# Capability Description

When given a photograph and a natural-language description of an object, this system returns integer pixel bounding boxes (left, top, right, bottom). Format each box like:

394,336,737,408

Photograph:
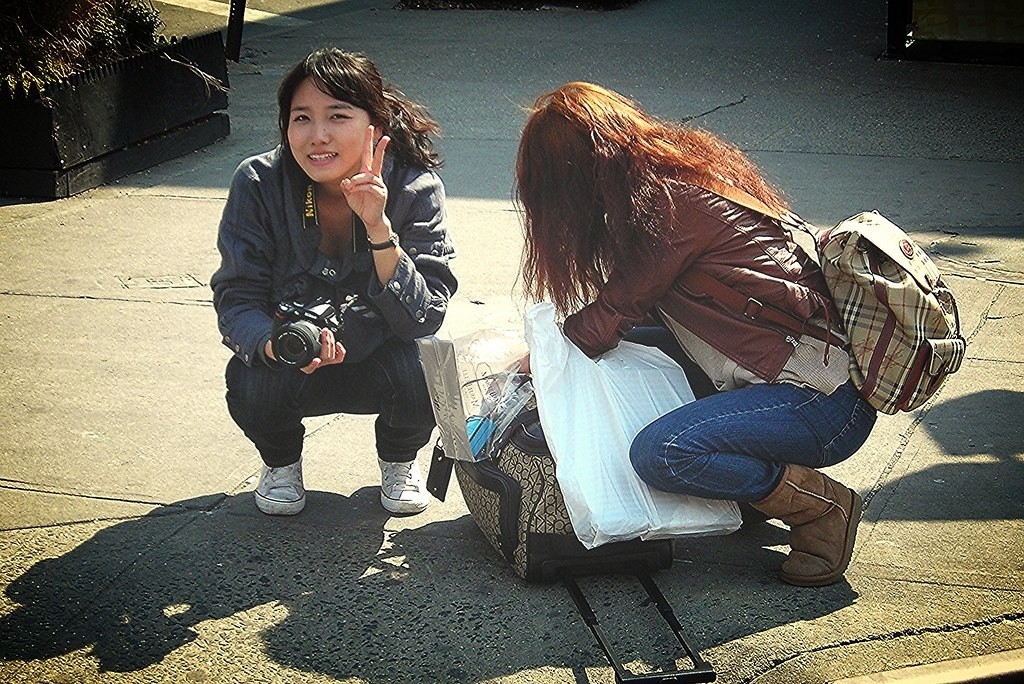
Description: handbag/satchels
524,298,743,551
415,324,533,461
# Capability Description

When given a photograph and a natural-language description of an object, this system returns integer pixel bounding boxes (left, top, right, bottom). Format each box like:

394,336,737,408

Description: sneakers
377,456,430,514
255,452,306,515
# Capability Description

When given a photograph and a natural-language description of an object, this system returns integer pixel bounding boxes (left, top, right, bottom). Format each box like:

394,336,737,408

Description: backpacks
658,167,967,415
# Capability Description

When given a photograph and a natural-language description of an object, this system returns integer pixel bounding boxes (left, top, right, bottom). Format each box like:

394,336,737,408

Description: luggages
453,360,673,582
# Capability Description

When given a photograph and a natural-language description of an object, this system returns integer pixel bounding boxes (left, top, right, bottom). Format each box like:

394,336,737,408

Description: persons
514,82,874,586
209,49,457,513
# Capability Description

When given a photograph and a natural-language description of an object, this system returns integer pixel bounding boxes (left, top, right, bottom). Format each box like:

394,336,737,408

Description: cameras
270,290,349,371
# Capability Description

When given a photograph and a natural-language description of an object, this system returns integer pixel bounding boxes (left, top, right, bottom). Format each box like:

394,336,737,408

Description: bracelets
367,232,399,250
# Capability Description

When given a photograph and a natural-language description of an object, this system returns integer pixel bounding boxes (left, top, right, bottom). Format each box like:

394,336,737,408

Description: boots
747,463,862,588
